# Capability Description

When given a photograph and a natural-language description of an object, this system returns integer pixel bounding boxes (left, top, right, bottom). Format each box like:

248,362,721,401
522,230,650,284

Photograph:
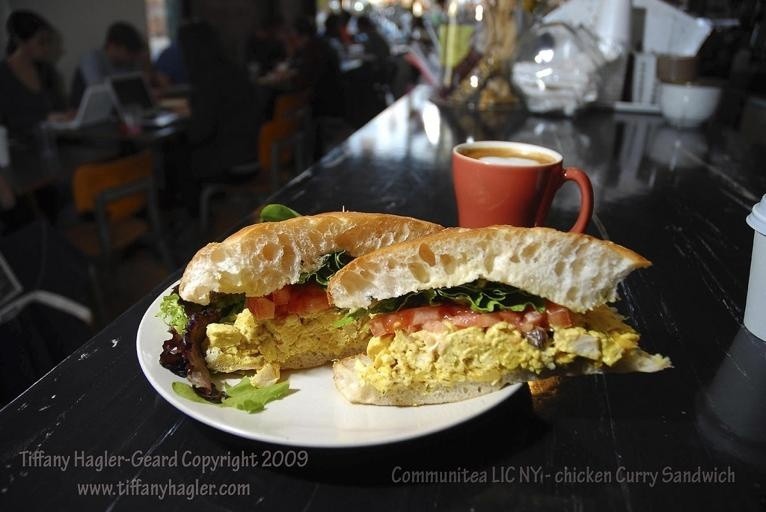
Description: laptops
72,84,111,123
105,73,154,119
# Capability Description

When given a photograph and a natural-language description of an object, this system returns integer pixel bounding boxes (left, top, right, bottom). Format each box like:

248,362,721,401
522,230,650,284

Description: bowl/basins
427,92,530,143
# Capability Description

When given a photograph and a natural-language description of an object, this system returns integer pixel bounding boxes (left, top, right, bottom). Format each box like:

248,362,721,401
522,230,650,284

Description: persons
0,9,436,409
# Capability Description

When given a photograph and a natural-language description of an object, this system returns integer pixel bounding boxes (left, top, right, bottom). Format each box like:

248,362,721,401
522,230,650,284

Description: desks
1,86,766,511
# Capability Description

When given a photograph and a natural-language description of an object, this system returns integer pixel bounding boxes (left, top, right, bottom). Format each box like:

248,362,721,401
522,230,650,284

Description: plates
133,277,528,450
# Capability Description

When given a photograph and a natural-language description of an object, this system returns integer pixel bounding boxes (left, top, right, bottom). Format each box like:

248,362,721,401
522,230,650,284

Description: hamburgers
157,205,675,415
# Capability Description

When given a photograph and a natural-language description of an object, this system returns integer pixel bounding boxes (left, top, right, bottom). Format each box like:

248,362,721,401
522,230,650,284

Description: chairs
61,150,168,310
197,86,311,243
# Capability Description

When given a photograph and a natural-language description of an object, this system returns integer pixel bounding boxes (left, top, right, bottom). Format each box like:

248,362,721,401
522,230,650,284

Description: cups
448,141,595,243
691,325,766,471
584,0,635,111
655,80,723,130
654,52,703,88
742,194,766,343
